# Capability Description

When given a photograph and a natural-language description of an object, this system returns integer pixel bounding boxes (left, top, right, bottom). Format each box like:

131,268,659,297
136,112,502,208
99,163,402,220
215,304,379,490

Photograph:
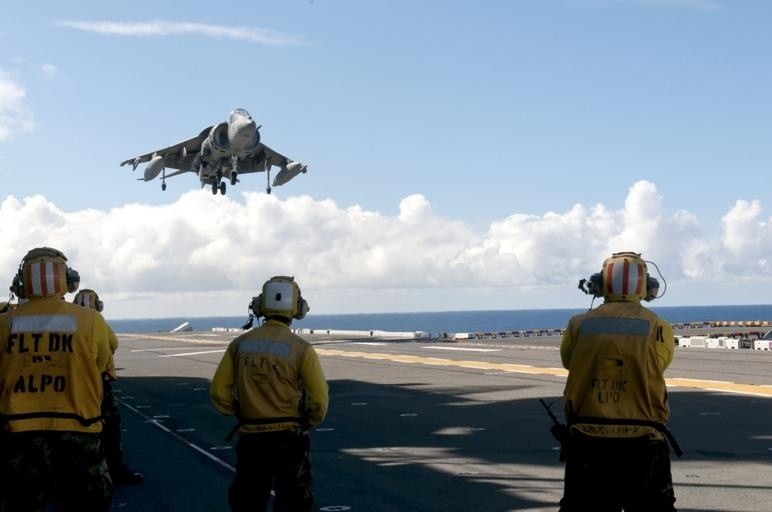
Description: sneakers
109,461,145,487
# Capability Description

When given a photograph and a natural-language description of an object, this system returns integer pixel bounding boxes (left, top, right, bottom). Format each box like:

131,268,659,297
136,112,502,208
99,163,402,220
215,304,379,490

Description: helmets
72,288,99,312
19,246,68,299
260,275,302,320
600,250,650,304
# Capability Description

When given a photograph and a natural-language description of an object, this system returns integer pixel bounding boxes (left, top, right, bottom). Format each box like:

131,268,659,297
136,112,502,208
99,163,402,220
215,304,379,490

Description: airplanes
120,108,308,195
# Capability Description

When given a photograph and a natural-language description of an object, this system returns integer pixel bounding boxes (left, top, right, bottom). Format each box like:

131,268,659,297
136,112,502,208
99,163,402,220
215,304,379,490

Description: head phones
248,275,310,320
9,247,80,298
589,251,660,303
74,288,103,312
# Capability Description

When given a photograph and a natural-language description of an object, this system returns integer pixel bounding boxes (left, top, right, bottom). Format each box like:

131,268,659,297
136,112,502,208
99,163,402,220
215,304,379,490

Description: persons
72,287,145,483
554,249,683,512
1,245,119,511
208,274,332,510
